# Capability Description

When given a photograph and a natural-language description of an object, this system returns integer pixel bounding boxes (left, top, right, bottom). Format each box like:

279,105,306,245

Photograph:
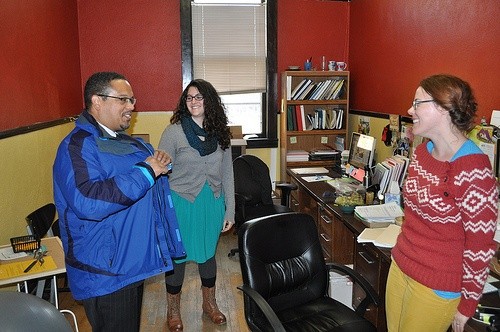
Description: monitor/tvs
347,131,376,171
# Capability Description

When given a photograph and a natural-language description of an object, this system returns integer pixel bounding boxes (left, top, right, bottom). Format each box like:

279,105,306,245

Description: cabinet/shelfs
286,164,403,332
280,70,349,197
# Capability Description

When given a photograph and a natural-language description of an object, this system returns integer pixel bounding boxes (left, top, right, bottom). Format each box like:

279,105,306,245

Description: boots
166,290,183,332
201,285,227,325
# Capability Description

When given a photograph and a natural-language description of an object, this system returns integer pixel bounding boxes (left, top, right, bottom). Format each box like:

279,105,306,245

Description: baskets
10,234,41,253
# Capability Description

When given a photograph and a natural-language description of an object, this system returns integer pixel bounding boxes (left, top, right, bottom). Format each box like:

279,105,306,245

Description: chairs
26,203,84,306
228,154,298,258
235,212,382,332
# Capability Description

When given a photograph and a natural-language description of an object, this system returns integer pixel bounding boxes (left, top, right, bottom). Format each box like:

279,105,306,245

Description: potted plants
335,192,364,214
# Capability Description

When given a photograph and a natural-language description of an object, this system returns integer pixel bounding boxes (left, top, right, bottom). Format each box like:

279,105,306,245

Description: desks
0,234,79,332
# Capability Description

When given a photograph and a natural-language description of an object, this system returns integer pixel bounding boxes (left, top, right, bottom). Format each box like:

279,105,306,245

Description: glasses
184,95,204,102
412,100,434,110
96,93,135,104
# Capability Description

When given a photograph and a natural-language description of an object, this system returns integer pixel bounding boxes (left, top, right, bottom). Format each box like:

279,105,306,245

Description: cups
304,62,312,71
336,61,347,71
328,61,336,72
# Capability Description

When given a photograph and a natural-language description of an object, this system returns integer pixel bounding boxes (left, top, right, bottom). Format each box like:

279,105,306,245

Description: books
286,76,409,196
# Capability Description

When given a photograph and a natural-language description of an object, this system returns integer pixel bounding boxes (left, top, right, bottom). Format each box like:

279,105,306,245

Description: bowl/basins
288,65,300,69
341,206,354,213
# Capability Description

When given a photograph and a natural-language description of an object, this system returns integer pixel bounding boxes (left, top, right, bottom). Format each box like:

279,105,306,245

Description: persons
384,74,499,332
158,80,237,332
52,72,186,332
